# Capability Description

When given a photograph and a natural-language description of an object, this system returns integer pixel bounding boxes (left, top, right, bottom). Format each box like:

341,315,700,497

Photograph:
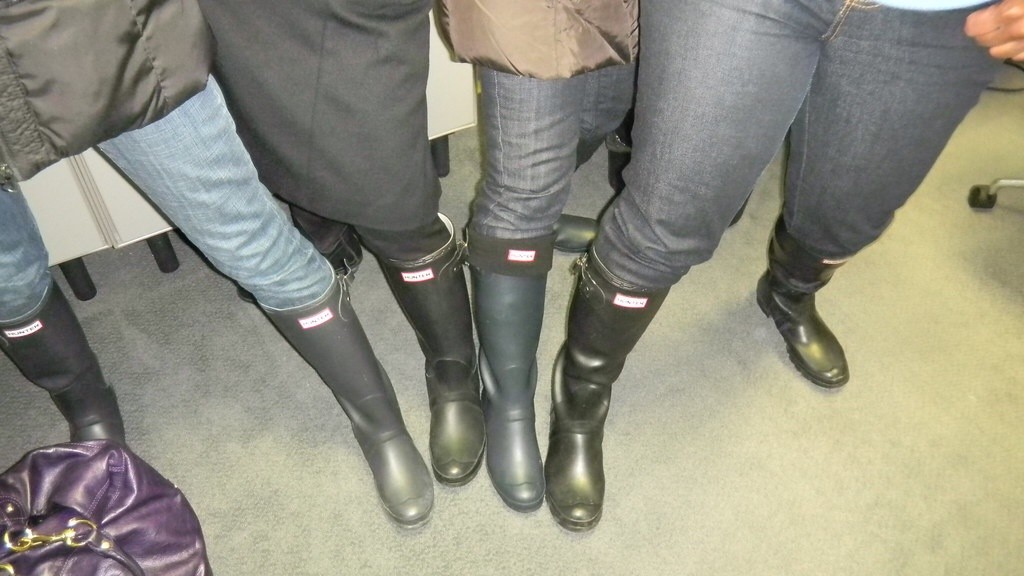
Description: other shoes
237,229,363,301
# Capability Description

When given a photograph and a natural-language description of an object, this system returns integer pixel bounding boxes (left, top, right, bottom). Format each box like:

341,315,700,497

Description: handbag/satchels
0,438,213,576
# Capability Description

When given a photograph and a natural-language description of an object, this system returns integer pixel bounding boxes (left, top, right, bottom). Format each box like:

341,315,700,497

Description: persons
545,0,1024,533
201,0,485,488
443,0,641,514
0,0,435,530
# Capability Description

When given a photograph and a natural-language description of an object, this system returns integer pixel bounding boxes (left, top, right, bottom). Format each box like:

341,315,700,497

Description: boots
552,138,605,252
0,272,124,444
462,226,558,513
374,211,485,487
257,255,435,530
544,240,671,532
756,201,849,388
